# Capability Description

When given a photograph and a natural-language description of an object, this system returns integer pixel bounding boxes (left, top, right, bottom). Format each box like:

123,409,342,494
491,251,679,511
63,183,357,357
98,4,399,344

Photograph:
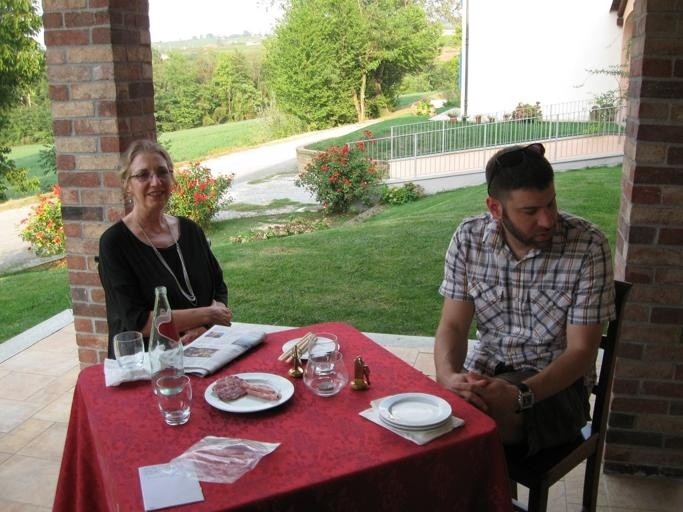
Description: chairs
511,280,633,510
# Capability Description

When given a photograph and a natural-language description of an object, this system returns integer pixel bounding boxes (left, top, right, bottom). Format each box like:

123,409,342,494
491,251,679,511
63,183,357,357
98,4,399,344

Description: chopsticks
278,332,316,363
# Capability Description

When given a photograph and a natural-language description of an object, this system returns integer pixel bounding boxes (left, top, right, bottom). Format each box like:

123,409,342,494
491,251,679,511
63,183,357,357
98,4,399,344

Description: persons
431,144,613,473
95,135,234,356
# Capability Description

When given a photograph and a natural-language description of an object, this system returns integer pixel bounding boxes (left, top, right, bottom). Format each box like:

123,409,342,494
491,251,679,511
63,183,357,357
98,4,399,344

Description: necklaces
129,208,199,306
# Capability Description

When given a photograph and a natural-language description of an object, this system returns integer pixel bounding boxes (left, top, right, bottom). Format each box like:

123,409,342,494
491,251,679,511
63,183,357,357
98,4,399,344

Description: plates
203,370,294,415
280,335,340,359
377,392,452,430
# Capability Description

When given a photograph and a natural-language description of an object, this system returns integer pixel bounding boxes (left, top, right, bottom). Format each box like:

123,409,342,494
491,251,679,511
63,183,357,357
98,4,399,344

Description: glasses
488,143,545,196
129,167,173,182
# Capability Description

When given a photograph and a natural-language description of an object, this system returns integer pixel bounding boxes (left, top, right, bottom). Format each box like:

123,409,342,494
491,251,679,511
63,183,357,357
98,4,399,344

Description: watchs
513,381,534,415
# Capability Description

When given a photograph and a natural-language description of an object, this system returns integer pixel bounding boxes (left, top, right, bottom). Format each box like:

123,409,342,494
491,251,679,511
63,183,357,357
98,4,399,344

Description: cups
307,331,339,354
302,350,349,398
155,374,195,428
111,328,145,371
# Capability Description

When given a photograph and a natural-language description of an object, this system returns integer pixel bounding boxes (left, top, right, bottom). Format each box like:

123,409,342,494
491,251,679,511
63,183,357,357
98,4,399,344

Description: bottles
148,284,184,397
